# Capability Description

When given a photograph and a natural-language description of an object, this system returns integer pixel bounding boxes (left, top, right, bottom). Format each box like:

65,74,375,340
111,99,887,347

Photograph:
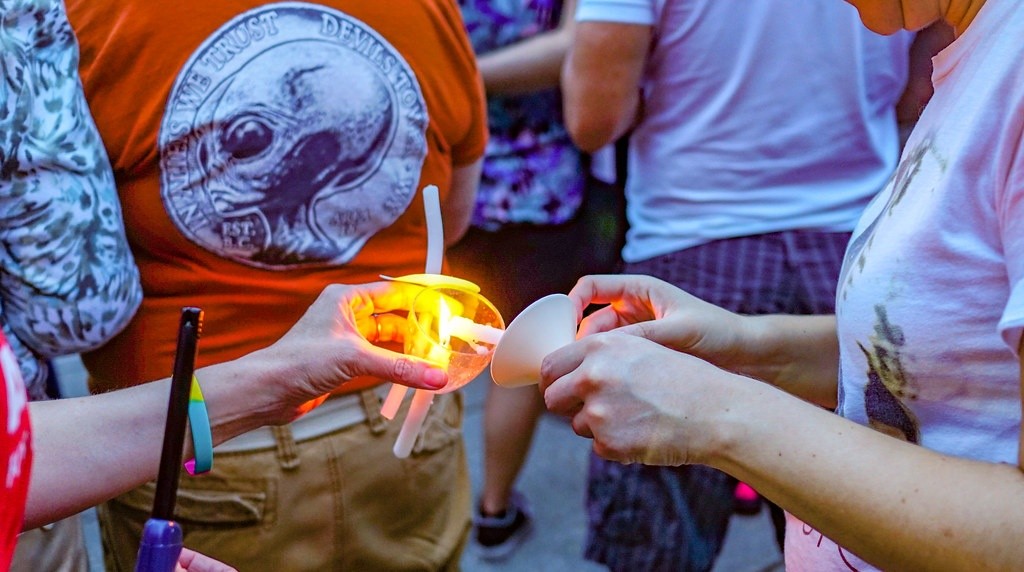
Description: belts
211,379,416,453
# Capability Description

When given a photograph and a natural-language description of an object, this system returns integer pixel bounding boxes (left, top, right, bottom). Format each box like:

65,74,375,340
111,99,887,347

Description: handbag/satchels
575,131,630,278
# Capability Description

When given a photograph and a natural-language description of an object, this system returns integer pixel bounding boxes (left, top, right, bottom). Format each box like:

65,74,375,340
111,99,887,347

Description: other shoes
737,495,761,515
474,490,535,559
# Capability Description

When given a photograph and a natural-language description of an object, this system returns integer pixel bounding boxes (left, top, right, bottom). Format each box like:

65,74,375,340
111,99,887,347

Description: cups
403,284,505,394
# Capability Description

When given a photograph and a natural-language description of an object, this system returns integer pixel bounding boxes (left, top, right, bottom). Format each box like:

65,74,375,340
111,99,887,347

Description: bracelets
182,366,215,478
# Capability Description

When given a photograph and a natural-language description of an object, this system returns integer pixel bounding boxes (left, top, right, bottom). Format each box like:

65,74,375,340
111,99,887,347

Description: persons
0,0,954,572
539,0,1024,572
